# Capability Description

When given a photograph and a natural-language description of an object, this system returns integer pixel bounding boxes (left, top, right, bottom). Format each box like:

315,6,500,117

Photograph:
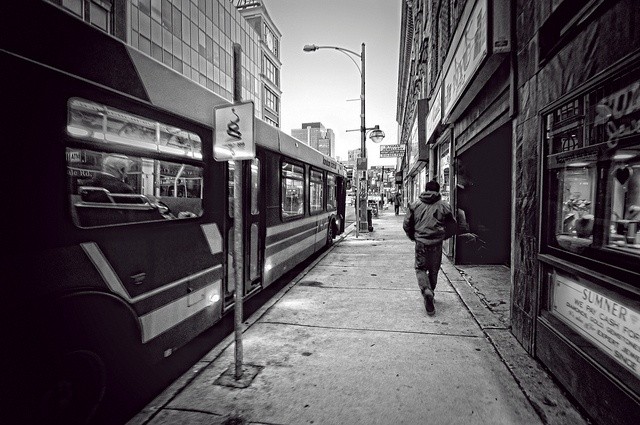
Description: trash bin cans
358,207,372,232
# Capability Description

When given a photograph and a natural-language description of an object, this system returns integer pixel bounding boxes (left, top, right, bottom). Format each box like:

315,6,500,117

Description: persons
84,154,144,203
392,194,402,214
403,181,456,315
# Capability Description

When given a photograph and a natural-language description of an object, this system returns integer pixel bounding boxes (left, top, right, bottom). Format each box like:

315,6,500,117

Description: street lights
303,41,386,234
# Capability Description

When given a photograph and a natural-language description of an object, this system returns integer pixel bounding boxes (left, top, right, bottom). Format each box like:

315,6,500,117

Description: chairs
73,187,166,227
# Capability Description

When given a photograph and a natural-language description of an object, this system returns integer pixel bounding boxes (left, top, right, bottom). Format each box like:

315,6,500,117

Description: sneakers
424,290,436,316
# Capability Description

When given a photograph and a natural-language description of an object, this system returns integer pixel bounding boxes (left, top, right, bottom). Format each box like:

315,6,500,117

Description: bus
0,0,353,424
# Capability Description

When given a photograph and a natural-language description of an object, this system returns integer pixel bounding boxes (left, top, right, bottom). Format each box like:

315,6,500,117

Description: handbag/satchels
398,201,402,206
